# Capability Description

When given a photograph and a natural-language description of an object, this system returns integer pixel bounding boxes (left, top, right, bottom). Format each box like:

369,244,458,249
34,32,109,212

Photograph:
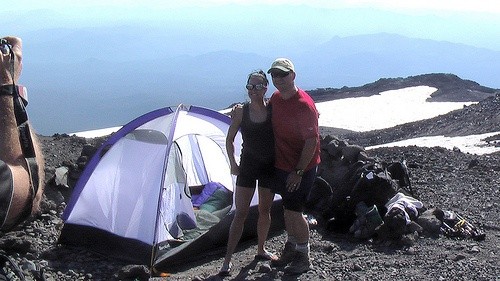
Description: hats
267,58,295,74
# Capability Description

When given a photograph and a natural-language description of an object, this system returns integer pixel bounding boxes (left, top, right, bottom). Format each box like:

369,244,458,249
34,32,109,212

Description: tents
57,104,285,268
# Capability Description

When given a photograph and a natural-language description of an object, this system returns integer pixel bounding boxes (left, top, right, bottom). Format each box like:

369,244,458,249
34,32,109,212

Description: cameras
0,39,12,54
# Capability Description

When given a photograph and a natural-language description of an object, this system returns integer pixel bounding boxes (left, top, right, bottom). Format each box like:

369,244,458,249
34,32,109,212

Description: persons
219,70,320,275
0,37,45,232
230,58,320,274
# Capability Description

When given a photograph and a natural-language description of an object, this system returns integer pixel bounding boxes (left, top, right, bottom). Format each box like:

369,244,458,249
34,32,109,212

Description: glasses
246,83,268,90
271,72,289,77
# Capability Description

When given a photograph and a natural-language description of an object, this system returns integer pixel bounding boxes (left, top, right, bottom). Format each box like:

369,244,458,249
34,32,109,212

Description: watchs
293,167,304,177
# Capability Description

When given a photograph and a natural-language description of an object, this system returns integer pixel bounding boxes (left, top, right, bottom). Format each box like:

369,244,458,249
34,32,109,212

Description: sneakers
272,242,312,276
255,249,279,262
219,262,232,276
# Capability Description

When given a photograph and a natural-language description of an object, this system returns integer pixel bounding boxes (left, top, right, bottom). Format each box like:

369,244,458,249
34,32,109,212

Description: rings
293,183,297,187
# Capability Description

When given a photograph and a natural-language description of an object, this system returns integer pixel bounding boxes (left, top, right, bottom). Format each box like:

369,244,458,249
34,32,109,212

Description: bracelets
0,84,28,107
